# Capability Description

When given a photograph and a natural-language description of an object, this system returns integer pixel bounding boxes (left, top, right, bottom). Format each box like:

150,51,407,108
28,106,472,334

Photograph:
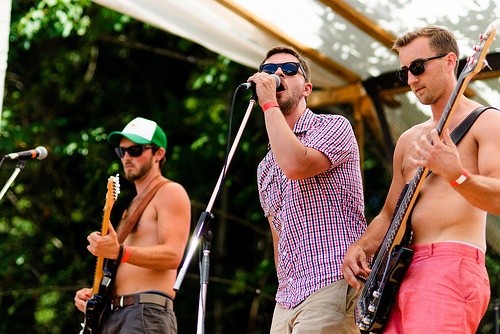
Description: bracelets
449,168,469,186
117,244,131,262
262,102,279,112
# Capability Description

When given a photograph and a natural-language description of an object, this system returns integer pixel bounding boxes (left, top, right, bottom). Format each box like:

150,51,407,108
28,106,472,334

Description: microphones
8,146,48,160
240,74,281,89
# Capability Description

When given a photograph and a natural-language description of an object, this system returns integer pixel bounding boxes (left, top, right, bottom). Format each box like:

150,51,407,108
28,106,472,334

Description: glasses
115,144,158,158
399,55,459,82
260,62,307,80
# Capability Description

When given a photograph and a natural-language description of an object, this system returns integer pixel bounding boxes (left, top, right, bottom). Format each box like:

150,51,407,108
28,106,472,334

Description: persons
246,46,374,334
73,116,191,334
342,26,500,334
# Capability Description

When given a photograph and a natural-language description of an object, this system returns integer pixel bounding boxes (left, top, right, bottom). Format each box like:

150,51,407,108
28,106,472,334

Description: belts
108,294,173,310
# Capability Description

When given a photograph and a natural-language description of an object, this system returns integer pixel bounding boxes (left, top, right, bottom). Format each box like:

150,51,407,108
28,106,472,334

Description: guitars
79,174,123,334
354,19,500,334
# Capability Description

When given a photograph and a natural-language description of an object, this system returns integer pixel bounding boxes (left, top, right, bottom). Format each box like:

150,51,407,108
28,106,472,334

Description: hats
108,117,167,150
